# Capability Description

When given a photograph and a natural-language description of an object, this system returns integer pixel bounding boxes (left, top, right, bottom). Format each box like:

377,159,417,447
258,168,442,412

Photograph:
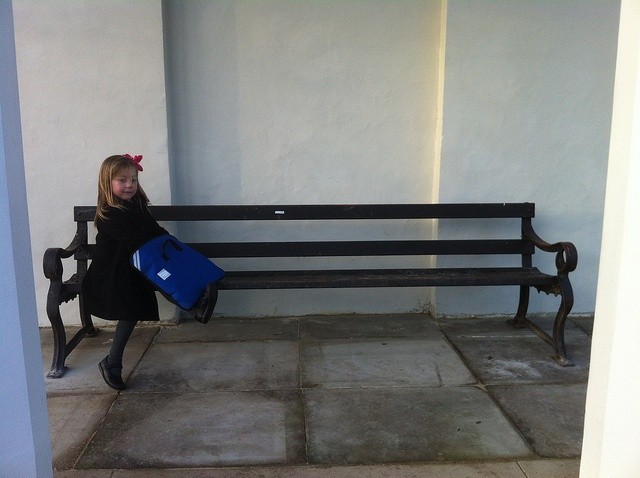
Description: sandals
193,281,219,324
99,354,126,390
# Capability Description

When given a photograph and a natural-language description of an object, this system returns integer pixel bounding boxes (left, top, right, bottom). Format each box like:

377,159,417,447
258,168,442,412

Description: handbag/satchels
129,233,225,310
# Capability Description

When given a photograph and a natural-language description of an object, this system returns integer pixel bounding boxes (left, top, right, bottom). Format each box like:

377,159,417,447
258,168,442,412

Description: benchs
43,202,578,378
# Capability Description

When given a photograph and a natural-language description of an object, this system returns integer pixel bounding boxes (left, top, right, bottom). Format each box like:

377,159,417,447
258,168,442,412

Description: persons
80,153,218,391
